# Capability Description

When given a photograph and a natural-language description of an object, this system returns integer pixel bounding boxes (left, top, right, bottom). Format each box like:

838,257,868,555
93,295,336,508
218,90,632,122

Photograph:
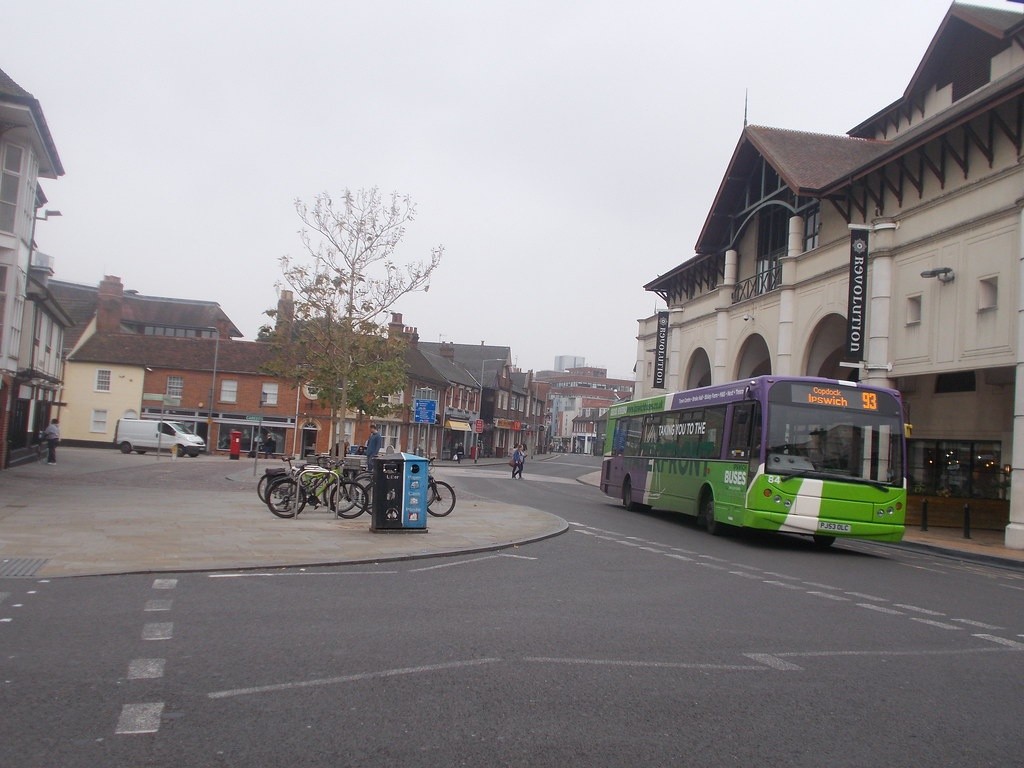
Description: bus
598,375,914,548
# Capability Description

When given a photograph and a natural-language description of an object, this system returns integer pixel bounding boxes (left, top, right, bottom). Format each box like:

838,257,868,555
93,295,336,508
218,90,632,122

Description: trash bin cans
370,452,429,533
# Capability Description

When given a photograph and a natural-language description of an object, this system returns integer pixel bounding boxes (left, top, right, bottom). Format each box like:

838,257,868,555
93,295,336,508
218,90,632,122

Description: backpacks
508,450,520,467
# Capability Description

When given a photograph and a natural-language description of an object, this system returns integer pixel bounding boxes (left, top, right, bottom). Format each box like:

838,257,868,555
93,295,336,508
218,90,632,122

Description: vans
115,418,206,458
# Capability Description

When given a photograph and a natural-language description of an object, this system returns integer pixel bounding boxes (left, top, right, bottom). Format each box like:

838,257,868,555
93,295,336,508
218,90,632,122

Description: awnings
445,419,471,431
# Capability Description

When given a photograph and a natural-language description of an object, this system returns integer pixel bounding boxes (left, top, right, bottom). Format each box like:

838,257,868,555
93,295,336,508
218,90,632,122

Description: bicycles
257,450,456,519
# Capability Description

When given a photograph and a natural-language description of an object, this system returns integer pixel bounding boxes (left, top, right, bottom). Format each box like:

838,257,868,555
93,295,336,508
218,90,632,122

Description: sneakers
47,460,56,465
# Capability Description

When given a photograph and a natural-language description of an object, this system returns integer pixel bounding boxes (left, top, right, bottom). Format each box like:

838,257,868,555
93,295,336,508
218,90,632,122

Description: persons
264,433,273,459
44,419,59,466
513,444,528,479
512,442,519,479
355,446,366,455
366,424,381,470
452,442,464,464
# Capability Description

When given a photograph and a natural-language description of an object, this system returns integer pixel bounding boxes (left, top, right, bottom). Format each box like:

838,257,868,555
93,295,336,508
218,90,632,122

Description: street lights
205,325,220,453
473,358,509,464
